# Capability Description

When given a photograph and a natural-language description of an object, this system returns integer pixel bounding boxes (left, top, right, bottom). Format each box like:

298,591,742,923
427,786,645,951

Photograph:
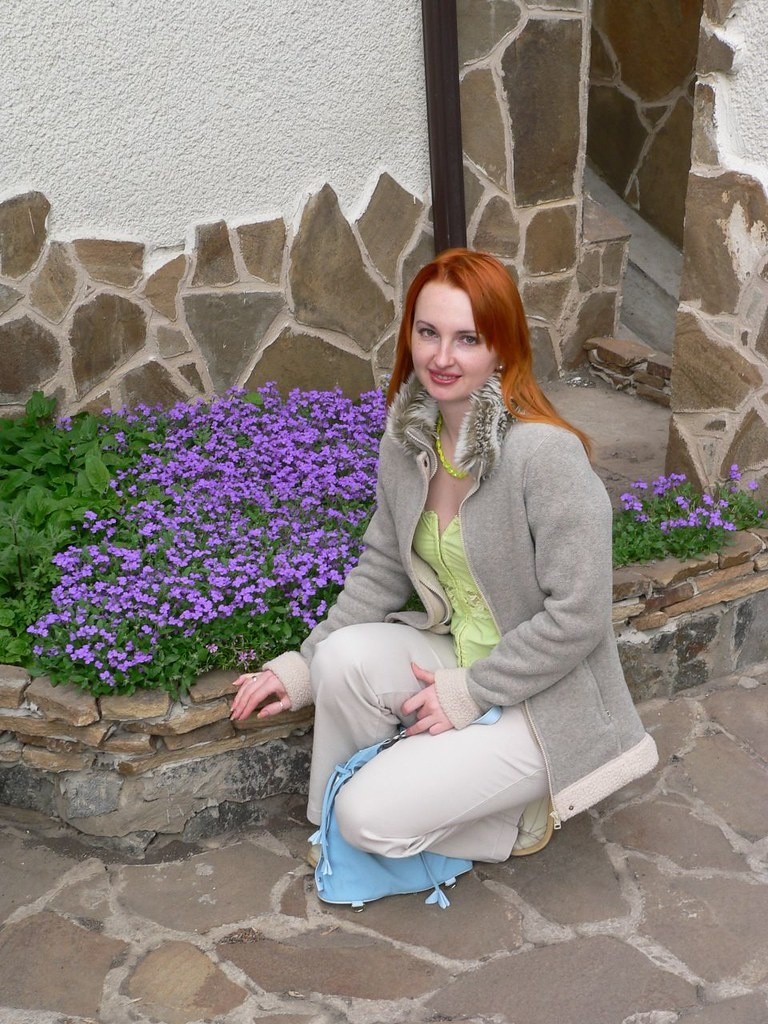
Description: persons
228,248,660,869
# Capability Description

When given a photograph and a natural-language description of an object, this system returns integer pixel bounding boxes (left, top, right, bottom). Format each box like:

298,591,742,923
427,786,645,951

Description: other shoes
510,795,555,856
308,843,322,868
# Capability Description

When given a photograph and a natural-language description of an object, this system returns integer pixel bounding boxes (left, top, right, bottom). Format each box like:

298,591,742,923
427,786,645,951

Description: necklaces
432,414,474,479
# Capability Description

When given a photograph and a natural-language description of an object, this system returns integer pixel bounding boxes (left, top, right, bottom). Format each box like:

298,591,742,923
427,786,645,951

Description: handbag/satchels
308,737,474,913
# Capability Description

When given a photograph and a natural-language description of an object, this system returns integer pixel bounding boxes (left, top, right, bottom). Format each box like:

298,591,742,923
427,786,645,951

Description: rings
251,675,259,682
279,699,286,711
